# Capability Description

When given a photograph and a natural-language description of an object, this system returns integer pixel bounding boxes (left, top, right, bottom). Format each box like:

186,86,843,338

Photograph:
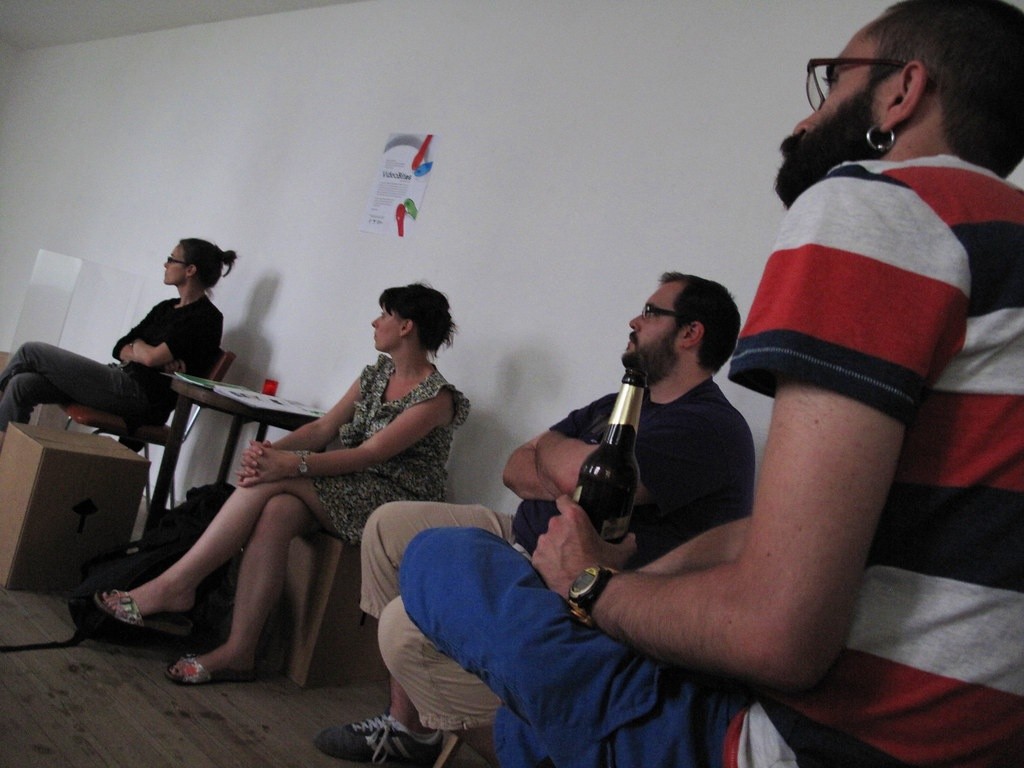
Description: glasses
641,303,676,319
806,57,937,112
167,257,199,273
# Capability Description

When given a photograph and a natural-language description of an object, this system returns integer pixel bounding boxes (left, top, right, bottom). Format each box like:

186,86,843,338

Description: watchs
298,452,308,478
568,563,622,628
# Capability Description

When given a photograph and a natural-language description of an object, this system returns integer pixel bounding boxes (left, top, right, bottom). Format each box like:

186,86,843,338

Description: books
174,371,326,418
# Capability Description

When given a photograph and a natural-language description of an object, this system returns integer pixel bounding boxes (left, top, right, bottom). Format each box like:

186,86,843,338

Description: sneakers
314,713,443,768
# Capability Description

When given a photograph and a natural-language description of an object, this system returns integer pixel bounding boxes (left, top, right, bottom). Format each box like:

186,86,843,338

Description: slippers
164,653,259,685
94,589,194,634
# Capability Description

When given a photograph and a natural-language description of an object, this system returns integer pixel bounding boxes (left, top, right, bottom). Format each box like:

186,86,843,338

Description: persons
0,238,237,447
94,283,470,684
315,271,756,768
398,0,1024,768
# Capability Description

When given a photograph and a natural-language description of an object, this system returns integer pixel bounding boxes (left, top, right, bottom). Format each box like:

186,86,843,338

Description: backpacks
158,481,236,553
70,519,236,651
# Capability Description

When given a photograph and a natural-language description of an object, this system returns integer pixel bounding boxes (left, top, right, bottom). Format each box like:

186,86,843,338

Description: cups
262,378,279,396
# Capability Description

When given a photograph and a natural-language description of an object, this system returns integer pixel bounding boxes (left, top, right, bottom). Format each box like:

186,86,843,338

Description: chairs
59,347,237,514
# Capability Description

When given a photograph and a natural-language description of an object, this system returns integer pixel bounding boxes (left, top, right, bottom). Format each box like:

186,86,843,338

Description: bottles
569,369,651,545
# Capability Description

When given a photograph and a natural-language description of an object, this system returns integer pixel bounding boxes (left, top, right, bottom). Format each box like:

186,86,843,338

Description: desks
170,378,319,485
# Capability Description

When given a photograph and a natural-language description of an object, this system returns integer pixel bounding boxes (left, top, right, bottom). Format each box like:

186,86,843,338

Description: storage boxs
0,420,152,592
215,526,389,687
